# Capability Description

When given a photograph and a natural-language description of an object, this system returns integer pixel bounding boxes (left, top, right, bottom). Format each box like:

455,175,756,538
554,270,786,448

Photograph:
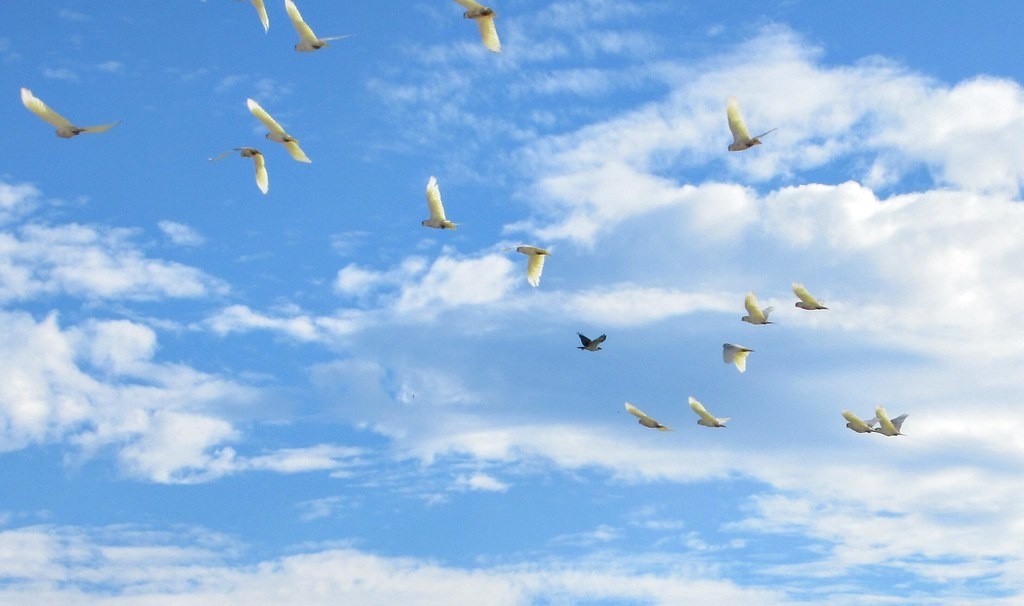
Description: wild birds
792,279,830,311
284,0,353,53
726,95,778,151
624,402,677,432
450,0,502,54
500,244,552,288
722,343,756,374
742,286,776,325
576,331,608,352
841,403,909,438
421,175,457,231
207,97,312,195
688,395,732,429
250,0,270,34
20,87,123,138
